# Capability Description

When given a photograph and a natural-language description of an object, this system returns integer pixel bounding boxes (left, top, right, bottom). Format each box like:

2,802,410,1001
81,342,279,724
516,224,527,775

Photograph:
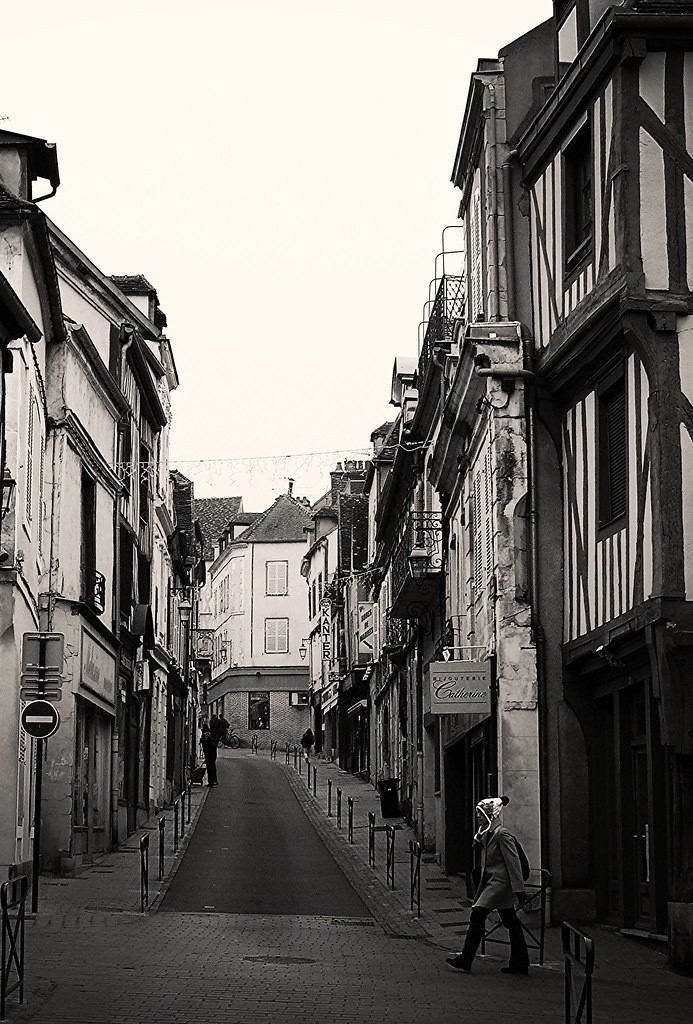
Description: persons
300,728,314,764
445,796,530,975
200,714,229,787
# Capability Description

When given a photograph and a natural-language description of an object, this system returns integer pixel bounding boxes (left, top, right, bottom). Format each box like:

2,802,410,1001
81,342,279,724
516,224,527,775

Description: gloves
515,891,528,905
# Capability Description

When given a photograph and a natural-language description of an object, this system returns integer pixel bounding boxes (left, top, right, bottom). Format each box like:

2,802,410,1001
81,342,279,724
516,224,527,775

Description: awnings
421,657,491,732
347,700,367,716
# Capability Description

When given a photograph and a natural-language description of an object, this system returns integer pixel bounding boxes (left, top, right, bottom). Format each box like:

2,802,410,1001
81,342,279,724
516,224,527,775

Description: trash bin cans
377,777,401,819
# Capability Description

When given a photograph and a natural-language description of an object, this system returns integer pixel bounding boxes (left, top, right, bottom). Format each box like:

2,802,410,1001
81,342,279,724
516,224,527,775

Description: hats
474,796,510,842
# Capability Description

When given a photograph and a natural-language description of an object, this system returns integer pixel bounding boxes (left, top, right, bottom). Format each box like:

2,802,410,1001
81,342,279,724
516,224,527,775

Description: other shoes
446,956,471,971
501,966,529,974
305,755,308,759
225,745,228,748
205,782,218,787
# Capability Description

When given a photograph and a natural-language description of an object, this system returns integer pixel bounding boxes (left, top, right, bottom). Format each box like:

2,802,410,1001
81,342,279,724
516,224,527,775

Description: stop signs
20,699,58,740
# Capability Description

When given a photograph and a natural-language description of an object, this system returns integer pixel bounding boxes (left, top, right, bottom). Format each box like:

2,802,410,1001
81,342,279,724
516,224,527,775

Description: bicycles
218,729,241,750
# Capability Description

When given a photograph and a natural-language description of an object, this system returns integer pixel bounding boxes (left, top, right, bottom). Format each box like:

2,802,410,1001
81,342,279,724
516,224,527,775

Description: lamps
219,641,229,660
298,638,310,660
406,526,448,586
169,588,193,628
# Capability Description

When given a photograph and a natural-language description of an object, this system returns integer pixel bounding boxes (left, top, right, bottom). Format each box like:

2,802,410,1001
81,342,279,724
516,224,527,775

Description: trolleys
190,761,207,786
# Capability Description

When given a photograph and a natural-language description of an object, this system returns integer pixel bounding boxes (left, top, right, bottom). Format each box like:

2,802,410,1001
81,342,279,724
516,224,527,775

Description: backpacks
498,828,530,881
